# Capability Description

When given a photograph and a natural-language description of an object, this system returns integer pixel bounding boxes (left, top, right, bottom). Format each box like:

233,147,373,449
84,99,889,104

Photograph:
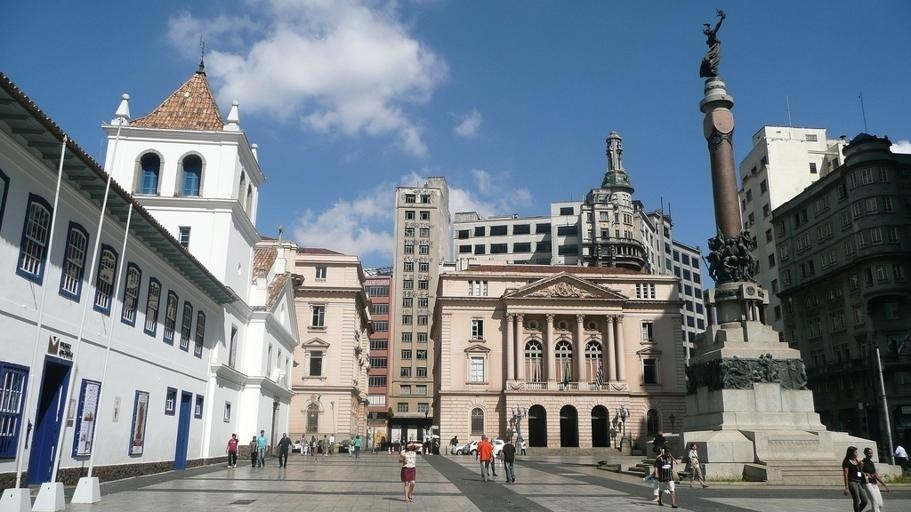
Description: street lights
372,411,378,454
616,404,633,456
331,399,337,445
511,404,527,455
859,400,871,438
425,408,430,436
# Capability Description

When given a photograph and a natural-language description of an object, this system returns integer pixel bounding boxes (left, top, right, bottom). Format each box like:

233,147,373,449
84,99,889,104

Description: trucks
880,398,911,446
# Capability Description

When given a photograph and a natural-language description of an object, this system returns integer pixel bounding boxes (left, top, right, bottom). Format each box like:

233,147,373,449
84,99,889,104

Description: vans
464,439,506,455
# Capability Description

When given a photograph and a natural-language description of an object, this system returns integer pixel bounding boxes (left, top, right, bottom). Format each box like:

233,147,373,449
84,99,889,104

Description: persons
298,433,363,465
276,433,295,468
256,430,269,468
687,442,710,489
397,440,424,505
249,436,258,467
858,446,889,512
645,445,680,509
893,443,909,472
474,433,529,483
424,438,440,454
448,435,459,455
698,17,723,77
224,432,239,468
705,228,756,280
842,446,869,512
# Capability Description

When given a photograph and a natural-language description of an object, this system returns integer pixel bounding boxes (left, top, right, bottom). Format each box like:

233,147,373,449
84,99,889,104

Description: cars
318,439,331,451
407,440,424,452
456,441,466,454
339,439,351,452
293,440,308,451
